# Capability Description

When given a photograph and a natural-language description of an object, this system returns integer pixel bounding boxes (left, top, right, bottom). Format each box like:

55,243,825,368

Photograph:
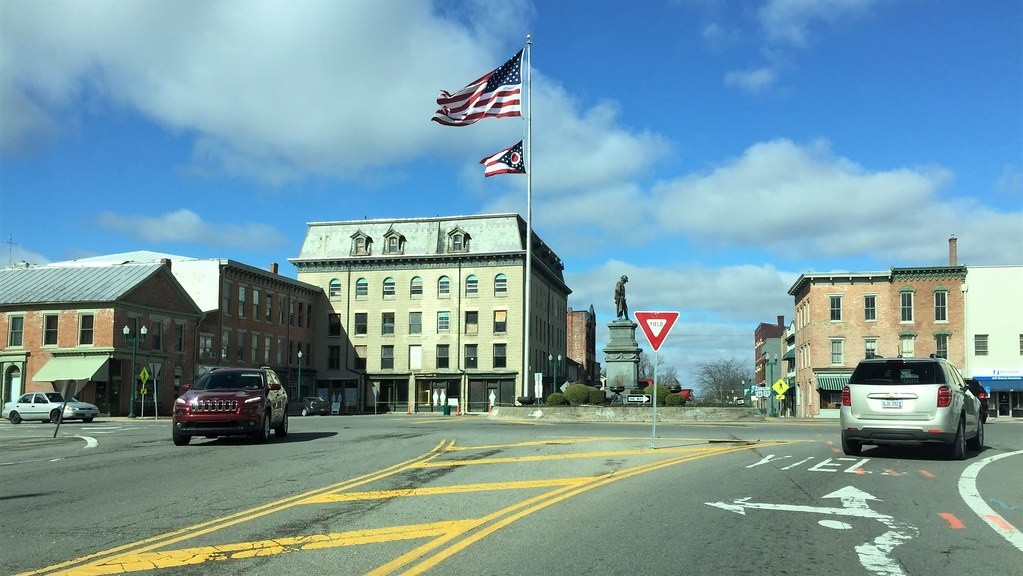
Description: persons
614,274,630,320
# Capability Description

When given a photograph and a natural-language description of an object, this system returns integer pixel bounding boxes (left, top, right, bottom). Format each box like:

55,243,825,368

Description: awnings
781,347,795,360
817,376,851,390
32,354,111,382
978,381,1023,393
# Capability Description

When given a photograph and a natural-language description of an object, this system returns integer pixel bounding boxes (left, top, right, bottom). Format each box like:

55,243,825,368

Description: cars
2,391,101,425
287,396,330,416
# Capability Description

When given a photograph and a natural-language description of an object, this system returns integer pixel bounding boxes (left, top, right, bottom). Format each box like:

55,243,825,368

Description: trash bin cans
442,402,451,416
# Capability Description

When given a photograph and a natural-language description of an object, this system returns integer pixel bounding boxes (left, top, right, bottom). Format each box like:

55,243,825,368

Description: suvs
838,353,985,461
171,366,290,446
963,378,989,423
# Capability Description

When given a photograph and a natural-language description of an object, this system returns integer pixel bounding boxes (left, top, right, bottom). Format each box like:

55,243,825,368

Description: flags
479,138,527,177
430,47,526,127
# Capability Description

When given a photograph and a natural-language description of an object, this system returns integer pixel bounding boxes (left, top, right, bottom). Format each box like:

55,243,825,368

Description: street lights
741,380,746,406
731,389,734,407
763,352,779,417
749,375,753,407
122,325,148,420
297,350,304,401
549,354,561,393
